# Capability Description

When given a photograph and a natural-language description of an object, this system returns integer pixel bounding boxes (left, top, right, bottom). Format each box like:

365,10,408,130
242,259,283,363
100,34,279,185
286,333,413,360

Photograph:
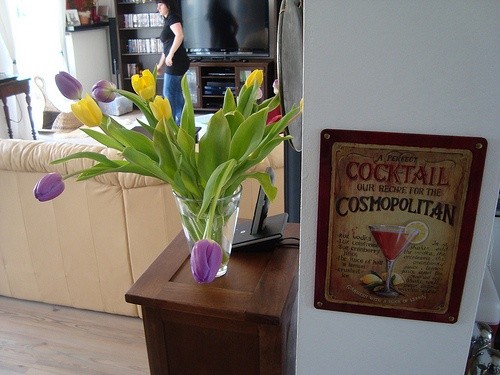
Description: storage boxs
98,95,134,116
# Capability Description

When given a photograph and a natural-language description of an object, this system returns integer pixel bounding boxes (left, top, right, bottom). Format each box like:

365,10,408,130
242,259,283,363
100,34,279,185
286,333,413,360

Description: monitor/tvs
178,0,277,62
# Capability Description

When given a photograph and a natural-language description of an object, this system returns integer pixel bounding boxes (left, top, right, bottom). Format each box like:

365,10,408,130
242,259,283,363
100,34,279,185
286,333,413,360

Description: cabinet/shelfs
124,218,300,375
114,0,164,95
187,59,272,112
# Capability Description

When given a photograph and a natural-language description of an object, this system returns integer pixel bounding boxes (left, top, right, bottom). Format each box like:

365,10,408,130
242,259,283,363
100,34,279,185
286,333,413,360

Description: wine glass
369,224,421,297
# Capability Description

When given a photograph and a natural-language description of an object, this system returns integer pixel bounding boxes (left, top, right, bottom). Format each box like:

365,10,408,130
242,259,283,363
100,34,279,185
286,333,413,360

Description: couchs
0,137,283,317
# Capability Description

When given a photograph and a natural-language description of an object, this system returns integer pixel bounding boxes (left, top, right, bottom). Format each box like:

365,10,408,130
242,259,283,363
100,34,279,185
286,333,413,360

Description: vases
172,185,243,278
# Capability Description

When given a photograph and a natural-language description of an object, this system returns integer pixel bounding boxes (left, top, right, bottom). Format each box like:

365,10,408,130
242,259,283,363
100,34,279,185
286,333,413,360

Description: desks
0,78,37,140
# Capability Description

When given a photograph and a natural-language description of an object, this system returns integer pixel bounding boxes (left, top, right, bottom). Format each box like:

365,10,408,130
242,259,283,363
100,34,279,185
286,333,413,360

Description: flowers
33,69,303,283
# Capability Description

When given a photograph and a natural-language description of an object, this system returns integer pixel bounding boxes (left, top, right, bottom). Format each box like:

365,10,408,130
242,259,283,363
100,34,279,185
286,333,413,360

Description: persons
155,0,190,125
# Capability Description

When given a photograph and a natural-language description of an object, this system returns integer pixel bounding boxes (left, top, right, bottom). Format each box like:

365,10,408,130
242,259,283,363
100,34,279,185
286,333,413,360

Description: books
127,63,137,77
128,38,163,53
124,13,165,28
